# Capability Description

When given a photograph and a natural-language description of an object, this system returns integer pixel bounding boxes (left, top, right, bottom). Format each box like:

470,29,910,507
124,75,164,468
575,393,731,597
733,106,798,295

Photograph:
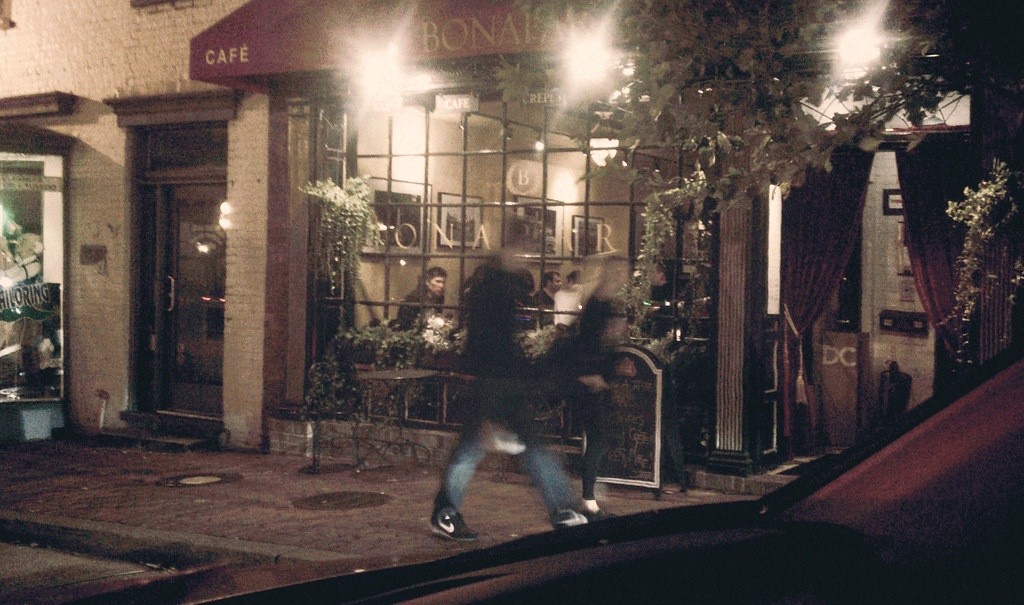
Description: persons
399,267,445,332
647,261,678,339
554,270,586,328
458,264,487,318
428,246,588,543
533,269,562,325
551,262,630,525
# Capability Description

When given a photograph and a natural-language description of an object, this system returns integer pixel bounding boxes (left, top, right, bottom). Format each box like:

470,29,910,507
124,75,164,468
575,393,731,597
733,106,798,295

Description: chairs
309,362,366,471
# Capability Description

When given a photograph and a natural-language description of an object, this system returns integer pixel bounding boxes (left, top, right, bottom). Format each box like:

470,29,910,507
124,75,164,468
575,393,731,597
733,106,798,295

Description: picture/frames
437,192,484,249
570,215,605,257
882,188,904,216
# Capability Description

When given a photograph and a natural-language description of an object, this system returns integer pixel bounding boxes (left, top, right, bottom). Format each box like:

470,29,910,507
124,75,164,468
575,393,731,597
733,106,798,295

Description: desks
353,367,441,470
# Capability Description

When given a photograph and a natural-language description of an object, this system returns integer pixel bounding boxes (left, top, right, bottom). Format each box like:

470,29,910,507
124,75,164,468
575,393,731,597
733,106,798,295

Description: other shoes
550,508,587,528
429,512,478,541
582,507,618,519
